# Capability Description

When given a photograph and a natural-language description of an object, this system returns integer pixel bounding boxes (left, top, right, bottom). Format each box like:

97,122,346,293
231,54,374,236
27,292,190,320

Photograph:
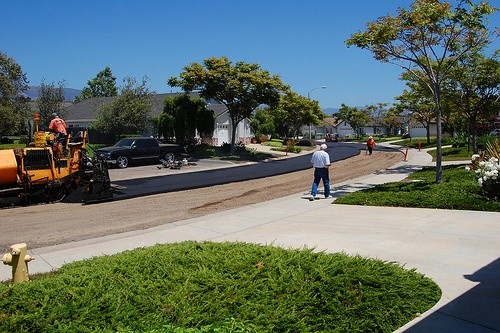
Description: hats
369,136,373,139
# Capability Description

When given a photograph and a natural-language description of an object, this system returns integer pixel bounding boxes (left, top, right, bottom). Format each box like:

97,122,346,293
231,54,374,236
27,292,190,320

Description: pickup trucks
97,136,180,168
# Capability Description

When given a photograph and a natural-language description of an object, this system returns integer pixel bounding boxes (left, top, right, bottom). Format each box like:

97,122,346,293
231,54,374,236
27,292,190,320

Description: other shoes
325,195,332,198
309,195,315,201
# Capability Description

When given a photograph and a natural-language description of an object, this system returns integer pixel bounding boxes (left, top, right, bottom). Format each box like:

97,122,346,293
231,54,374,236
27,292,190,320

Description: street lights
308,86,327,139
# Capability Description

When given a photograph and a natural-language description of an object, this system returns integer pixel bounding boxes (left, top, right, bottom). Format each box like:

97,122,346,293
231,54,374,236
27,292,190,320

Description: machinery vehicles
1,110,127,204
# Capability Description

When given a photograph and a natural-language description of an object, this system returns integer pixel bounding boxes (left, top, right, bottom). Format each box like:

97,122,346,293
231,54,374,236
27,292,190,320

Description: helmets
51,113,57,118
